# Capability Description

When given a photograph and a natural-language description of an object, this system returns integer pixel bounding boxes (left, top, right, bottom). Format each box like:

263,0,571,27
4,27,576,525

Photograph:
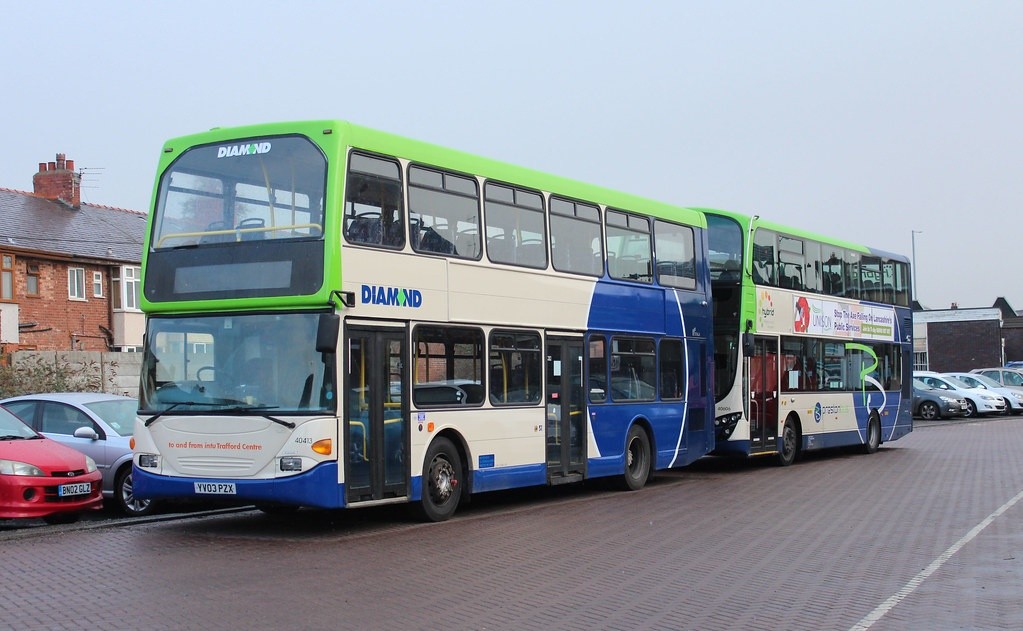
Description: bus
132,121,715,522
685,208,914,467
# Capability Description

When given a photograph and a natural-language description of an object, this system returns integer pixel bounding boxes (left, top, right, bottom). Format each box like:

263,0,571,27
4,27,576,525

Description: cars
945,372,1023,416
0,392,155,518
1005,360,1023,368
0,405,106,518
935,374,1008,418
914,378,968,420
970,369,1023,386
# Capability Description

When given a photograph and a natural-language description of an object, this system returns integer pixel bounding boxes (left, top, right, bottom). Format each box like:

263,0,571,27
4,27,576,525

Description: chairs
488,360,525,396
341,211,694,290
777,265,893,305
